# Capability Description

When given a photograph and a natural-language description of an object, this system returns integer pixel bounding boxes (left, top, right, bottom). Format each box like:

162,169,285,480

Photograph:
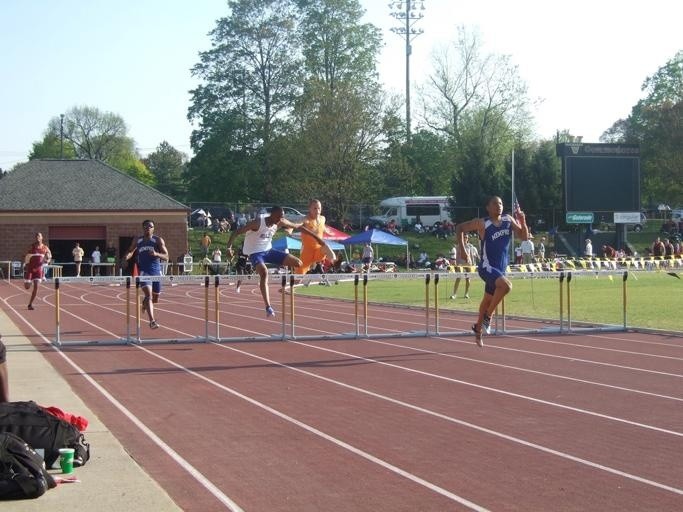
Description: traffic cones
132,264,139,277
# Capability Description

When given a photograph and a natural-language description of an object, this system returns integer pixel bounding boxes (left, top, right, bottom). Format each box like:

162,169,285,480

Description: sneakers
149,321,159,329
266,307,275,318
471,323,484,347
28,304,34,310
450,295,470,300
141,301,147,313
482,311,492,335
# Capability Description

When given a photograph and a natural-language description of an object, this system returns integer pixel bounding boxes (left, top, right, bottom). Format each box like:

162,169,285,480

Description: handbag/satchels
0,400,91,471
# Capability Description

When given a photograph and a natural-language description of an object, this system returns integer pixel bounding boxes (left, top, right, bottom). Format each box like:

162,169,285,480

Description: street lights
386,0,431,140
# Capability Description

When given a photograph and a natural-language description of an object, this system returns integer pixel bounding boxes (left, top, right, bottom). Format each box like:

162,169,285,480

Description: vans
188,206,237,232
602,211,648,232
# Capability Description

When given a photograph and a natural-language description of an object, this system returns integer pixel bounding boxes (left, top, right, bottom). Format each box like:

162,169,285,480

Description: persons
228,206,310,317
295,199,332,284
1,340,9,402
23,233,51,310
651,218,683,257
449,234,482,299
72,243,84,278
123,220,168,329
515,220,625,264
195,214,247,293
277,218,456,273
91,246,101,276
455,195,528,347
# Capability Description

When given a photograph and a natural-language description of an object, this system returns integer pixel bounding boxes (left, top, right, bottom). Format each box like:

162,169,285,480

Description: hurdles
135,274,211,345
434,272,497,337
53,275,133,347
291,271,359,341
214,275,289,342
568,270,627,334
501,272,564,333
363,272,431,339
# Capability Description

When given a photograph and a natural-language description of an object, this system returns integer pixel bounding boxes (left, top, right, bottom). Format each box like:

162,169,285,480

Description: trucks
368,196,455,233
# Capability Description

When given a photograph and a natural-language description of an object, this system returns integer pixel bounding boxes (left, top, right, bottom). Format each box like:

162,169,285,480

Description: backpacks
0,432,57,502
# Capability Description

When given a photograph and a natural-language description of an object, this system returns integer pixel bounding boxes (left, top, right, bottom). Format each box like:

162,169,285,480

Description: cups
57,447,75,472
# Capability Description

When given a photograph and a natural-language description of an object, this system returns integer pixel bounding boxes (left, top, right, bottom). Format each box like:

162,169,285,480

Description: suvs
256,207,305,223
